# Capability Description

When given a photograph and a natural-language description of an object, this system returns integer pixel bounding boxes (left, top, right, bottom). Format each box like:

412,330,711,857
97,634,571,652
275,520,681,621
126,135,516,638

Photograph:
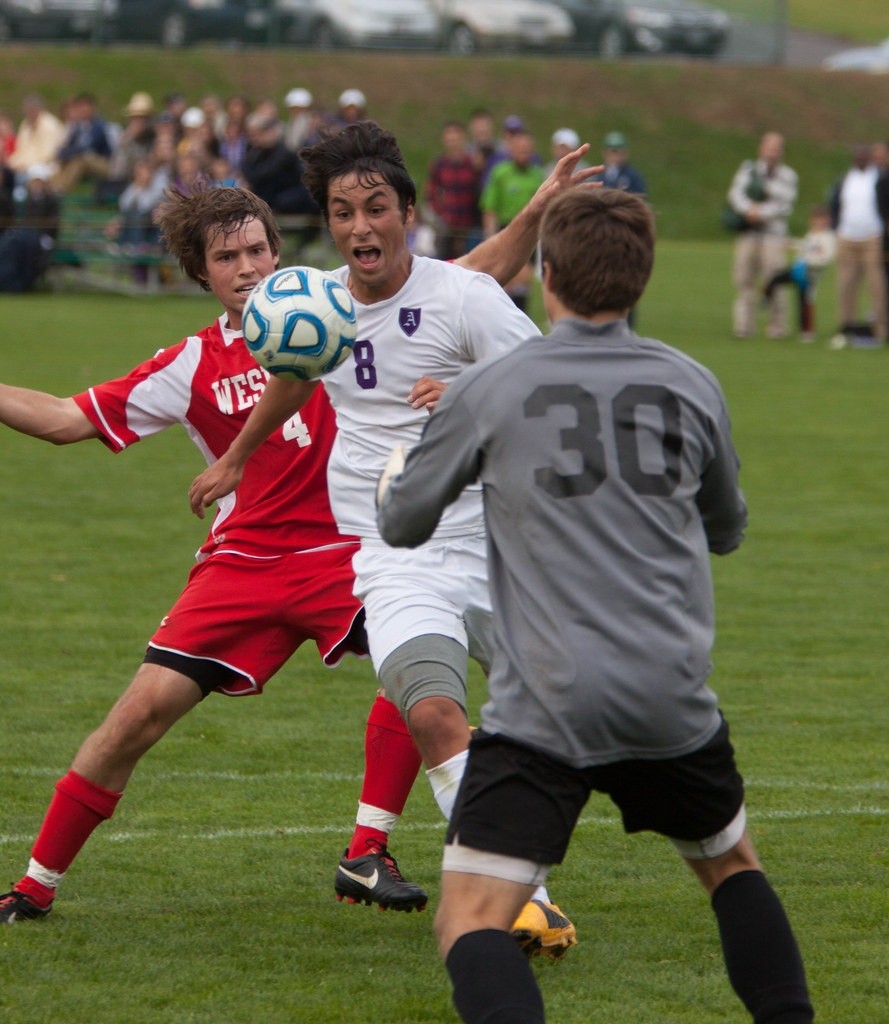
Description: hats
124,91,152,117
339,88,366,110
284,88,314,109
552,126,578,150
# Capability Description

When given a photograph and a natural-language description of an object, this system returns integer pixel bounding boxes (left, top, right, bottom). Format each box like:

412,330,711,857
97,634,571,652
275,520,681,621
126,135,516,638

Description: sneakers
508,899,577,960
336,839,428,915
0,891,53,923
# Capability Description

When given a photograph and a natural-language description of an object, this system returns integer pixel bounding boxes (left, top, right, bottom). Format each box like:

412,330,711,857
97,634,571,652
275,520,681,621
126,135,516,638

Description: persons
726,130,889,347
425,109,649,307
369,180,821,1024
0,139,635,926
0,89,367,295
188,128,580,951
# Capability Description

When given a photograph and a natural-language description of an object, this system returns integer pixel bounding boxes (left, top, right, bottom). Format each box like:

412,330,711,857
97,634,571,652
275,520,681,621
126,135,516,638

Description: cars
0,0,739,58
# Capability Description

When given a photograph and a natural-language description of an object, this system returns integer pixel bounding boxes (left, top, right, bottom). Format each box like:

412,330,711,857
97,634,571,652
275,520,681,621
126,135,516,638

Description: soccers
240,264,360,384
